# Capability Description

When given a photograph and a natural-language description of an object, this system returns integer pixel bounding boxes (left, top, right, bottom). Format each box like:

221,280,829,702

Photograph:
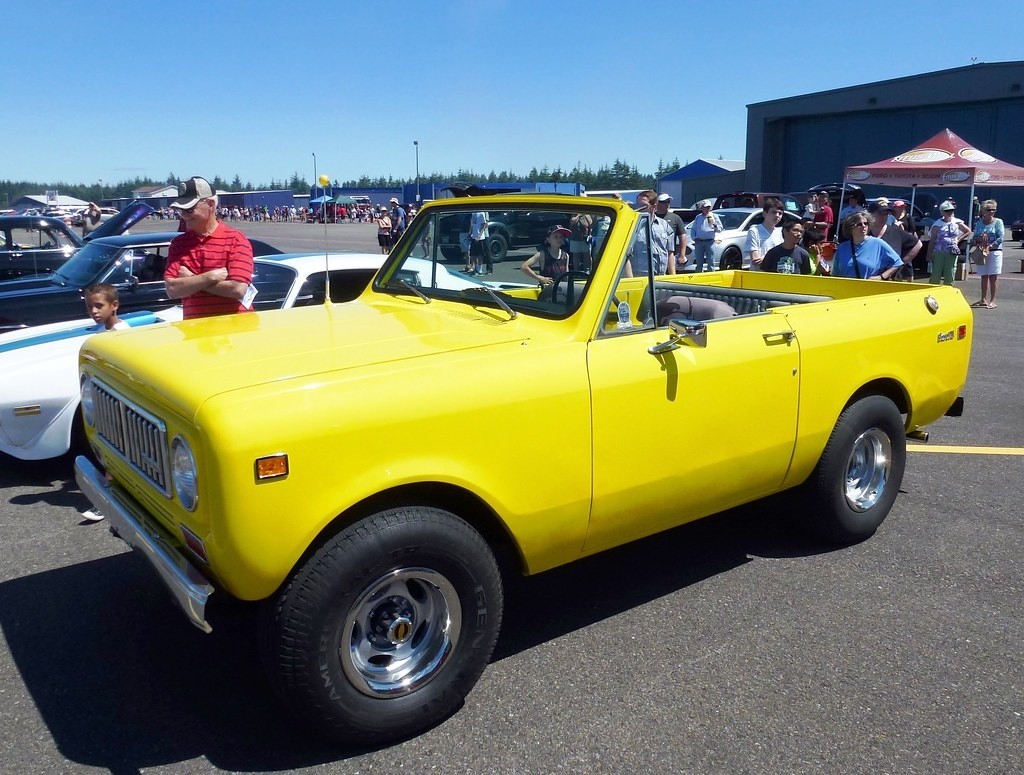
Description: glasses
850,222,868,227
173,199,209,216
986,209,996,212
660,200,670,203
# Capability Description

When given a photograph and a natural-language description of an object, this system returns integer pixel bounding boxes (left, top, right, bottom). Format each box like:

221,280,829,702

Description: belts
700,238,714,241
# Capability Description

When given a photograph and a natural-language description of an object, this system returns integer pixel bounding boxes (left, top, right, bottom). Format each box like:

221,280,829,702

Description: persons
461,212,494,277
743,188,924,282
691,200,723,273
696,203,701,209
925,198,971,287
521,225,572,290
970,197,1004,309
25,208,47,232
388,197,433,259
593,190,687,279
81,283,131,521
81,201,102,236
148,206,182,220
570,214,593,274
163,176,254,320
215,203,382,224
378,206,392,254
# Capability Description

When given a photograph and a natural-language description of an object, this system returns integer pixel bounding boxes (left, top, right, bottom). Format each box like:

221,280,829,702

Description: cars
19,201,120,232
0,250,536,471
435,206,604,265
0,232,290,338
661,179,941,278
0,200,156,285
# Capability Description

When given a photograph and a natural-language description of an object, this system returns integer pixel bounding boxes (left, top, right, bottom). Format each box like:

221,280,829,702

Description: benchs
637,280,834,322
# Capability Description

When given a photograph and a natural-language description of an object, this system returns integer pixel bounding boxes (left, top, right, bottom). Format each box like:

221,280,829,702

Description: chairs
140,253,163,282
537,282,585,306
652,297,737,327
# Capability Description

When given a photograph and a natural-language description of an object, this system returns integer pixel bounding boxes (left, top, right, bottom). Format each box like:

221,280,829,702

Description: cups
707,216,712,223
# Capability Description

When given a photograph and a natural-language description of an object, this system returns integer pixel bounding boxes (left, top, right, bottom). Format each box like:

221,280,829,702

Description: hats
545,225,572,237
170,176,216,209
390,198,399,205
943,204,956,211
381,207,387,211
702,200,711,207
657,193,673,202
868,201,894,214
893,200,907,207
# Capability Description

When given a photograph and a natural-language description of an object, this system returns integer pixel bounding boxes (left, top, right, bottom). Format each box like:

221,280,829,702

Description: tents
310,194,357,224
835,127,1024,280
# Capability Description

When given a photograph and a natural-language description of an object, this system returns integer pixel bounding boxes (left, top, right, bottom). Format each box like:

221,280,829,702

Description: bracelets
880,274,884,280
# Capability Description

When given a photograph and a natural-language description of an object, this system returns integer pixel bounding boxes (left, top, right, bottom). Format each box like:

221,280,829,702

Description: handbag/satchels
969,237,989,266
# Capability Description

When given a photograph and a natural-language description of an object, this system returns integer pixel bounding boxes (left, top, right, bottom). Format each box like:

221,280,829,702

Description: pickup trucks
67,186,977,756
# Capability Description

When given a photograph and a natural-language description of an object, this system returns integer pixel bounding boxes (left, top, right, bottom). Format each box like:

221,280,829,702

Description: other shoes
971,302,987,307
462,265,492,277
82,507,105,521
987,304,997,308
